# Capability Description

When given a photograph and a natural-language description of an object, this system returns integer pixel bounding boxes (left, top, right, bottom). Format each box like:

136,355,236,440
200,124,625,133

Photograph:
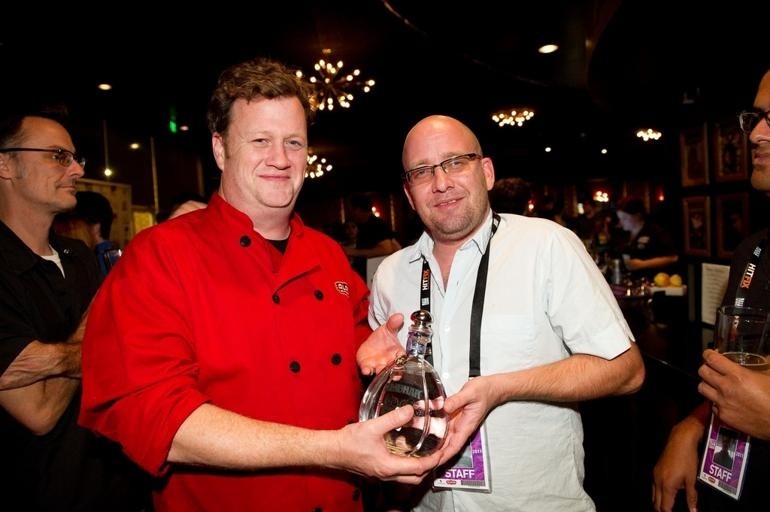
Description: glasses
401,153,482,185
738,109,770,133
0,147,87,170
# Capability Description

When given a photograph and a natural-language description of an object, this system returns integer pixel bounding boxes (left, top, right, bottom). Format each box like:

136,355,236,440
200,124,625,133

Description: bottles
357,311,448,459
609,258,623,286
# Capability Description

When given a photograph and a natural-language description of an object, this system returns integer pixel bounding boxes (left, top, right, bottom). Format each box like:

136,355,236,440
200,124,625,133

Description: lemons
654,272,670,287
670,274,681,287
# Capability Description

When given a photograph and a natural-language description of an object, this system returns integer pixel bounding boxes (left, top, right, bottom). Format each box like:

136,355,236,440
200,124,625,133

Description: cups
716,304,770,370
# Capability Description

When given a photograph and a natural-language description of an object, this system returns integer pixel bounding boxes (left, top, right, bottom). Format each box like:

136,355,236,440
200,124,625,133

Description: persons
156,192,208,222
365,113,645,512
651,68,769,512
76,58,446,512
1,113,150,512
315,179,681,283
55,193,120,278
713,433,734,468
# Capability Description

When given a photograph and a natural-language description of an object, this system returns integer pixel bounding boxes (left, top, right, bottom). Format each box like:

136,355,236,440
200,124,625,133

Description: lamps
296,58,377,112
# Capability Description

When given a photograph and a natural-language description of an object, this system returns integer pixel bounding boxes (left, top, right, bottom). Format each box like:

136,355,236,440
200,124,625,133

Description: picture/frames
716,192,750,259
682,196,712,258
714,116,751,183
679,122,710,188
